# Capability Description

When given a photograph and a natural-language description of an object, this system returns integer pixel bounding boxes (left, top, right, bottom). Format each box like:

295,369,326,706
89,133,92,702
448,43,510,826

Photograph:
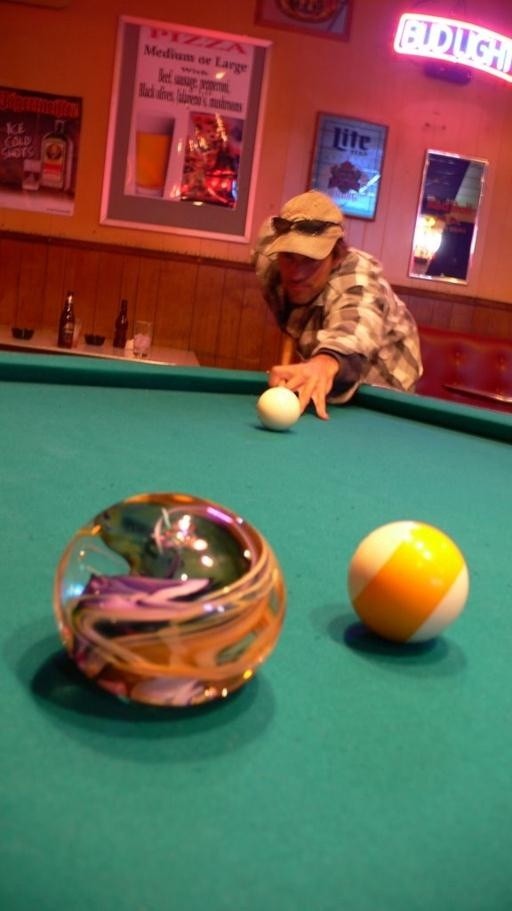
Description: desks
443,382,512,410
1,324,201,369
1,350,512,905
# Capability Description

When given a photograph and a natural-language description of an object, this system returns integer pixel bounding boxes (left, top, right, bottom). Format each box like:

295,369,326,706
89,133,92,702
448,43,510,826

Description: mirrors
406,147,489,286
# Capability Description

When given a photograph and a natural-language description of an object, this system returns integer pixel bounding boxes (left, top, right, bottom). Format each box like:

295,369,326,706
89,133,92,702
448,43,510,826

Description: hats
262,190,346,262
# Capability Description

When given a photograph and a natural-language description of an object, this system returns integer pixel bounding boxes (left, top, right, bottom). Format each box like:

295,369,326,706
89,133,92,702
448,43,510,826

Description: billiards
257,386,300,431
347,521,468,643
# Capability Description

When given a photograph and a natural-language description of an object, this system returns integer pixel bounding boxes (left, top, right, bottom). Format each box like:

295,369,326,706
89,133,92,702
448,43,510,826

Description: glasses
269,214,345,236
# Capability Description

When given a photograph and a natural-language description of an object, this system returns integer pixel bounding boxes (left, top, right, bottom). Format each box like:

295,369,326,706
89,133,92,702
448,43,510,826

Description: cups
22,160,42,192
132,321,152,360
73,318,84,349
133,110,177,198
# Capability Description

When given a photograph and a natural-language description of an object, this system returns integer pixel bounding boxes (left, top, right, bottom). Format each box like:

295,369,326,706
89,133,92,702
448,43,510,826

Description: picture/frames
308,112,390,222
253,1,354,46
99,13,273,245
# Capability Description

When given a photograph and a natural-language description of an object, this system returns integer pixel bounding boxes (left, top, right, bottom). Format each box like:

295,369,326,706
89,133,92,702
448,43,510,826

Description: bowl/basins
13,328,33,340
84,333,106,345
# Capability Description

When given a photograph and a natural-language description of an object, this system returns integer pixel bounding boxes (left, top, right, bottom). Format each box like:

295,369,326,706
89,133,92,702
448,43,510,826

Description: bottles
113,299,129,348
58,292,75,349
39,119,73,191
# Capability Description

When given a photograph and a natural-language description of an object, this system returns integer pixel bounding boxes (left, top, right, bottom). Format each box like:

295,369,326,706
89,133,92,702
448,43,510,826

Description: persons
249,188,424,421
